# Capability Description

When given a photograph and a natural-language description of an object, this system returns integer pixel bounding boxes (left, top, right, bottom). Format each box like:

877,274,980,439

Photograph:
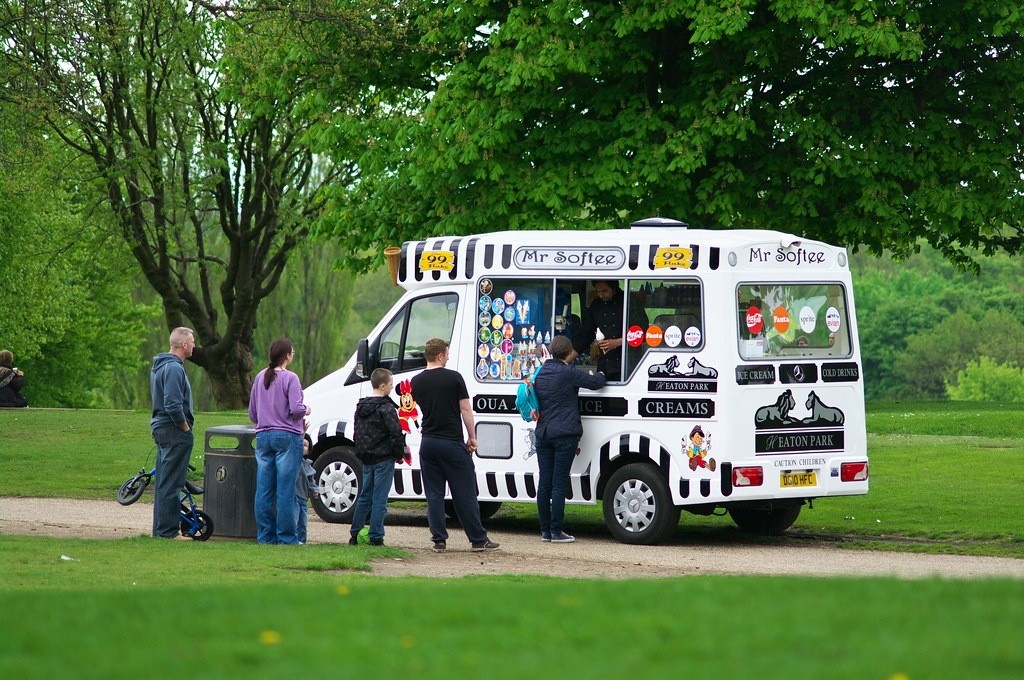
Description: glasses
289,351,295,355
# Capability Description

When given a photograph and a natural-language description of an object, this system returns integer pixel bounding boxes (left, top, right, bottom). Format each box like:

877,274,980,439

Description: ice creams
596,327,605,355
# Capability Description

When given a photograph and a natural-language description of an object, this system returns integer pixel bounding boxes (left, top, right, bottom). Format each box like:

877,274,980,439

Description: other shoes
367,538,385,546
349,534,359,545
172,535,193,542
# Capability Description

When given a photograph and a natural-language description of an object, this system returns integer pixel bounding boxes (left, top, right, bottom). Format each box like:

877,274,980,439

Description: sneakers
542,531,551,542
471,537,500,551
432,541,446,553
551,531,575,543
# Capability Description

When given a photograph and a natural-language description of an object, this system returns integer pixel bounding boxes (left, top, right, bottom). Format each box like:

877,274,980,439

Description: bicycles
117,446,213,541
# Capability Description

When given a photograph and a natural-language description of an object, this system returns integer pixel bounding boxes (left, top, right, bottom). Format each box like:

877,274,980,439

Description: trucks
299,217,872,543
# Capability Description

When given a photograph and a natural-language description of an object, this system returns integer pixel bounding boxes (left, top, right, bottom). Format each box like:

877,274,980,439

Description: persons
409,338,500,554
0,350,30,407
532,335,606,543
296,421,316,544
249,338,311,546
564,280,649,381
349,367,406,547
149,326,196,542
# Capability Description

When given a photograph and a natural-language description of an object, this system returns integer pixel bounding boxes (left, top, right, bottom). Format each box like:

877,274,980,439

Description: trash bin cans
203,425,258,538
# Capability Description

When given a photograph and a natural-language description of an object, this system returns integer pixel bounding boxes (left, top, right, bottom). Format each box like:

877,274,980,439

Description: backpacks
515,365,542,422
